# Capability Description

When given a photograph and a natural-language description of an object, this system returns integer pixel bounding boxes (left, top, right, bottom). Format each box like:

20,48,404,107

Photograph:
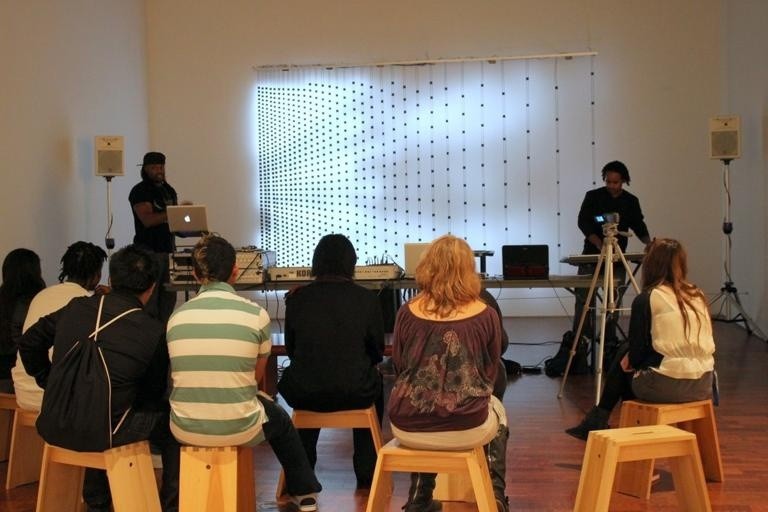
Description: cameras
594,211,620,224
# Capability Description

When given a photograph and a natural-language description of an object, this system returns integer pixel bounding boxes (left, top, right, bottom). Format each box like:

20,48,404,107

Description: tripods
706,159,768,341
558,237,641,406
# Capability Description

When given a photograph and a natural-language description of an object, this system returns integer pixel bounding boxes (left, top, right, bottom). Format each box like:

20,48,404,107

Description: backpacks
35,295,142,454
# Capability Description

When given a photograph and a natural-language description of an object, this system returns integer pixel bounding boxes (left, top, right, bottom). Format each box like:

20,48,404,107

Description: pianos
266,265,405,280
559,253,646,265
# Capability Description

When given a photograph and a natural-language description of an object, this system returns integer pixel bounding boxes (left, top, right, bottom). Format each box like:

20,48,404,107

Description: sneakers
290,489,321,512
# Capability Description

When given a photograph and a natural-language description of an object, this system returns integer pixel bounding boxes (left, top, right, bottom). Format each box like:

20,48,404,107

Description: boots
403,471,440,512
479,424,512,511
566,403,611,437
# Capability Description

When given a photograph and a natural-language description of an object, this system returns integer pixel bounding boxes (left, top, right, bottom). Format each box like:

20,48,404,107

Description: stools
177,444,257,512
365,436,497,512
272,405,394,500
33,440,162,512
612,395,725,500
571,422,712,511
0,392,18,462
4,406,44,490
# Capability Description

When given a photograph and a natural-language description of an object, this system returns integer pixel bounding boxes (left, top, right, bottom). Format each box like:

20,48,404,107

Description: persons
11,240,107,410
278,235,385,485
391,236,511,512
128,151,193,317
1,248,44,394
564,240,715,441
167,236,322,511
572,161,655,334
21,244,178,511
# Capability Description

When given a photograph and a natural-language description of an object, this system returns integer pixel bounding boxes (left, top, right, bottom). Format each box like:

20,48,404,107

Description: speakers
709,115,742,158
95,136,125,176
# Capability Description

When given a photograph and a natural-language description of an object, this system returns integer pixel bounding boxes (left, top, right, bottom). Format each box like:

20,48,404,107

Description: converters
522,366,540,373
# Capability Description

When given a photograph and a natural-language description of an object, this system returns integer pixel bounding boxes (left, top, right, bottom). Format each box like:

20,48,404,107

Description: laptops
503,245,548,280
166,205,209,233
405,243,431,279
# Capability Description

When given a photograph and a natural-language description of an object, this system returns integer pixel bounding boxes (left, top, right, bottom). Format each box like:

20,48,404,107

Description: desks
395,274,621,374
